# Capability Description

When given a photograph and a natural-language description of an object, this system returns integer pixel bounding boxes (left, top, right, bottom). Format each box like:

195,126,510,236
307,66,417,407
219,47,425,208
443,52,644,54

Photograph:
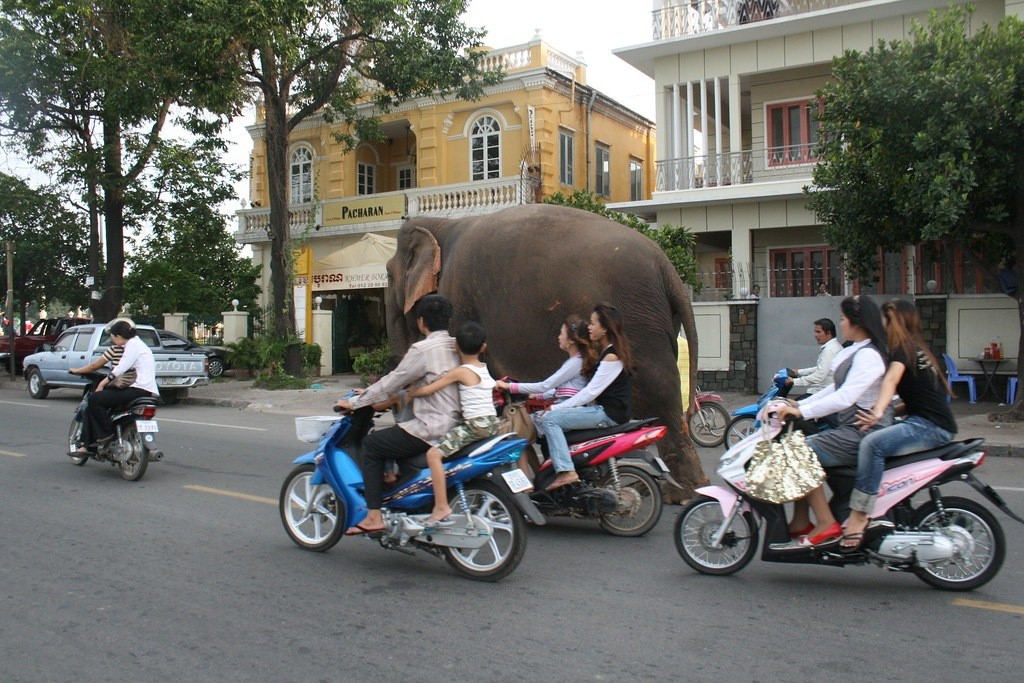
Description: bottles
984,347,990,359
992,343,1004,359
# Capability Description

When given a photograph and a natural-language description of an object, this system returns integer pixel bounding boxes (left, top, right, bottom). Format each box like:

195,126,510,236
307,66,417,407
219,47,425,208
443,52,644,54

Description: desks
967,358,1009,403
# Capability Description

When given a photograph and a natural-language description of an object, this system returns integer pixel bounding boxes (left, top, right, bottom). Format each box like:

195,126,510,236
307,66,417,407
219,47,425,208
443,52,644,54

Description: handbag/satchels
106,368,137,389
497,402,538,446
744,429,826,505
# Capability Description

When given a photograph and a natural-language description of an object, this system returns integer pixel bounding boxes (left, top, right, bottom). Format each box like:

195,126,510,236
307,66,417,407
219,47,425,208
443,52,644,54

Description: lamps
738,286,748,298
124,303,131,313
142,305,148,313
926,280,937,293
314,296,322,310
231,299,239,311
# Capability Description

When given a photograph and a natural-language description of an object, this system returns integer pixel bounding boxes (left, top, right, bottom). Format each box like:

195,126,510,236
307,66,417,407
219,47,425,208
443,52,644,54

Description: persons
332,293,500,535
839,299,958,548
494,315,599,493
69,318,160,452
785,318,845,436
540,301,637,492
775,293,894,548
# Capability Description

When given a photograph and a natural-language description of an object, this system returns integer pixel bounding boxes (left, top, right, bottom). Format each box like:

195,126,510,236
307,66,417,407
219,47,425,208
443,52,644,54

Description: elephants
385,203,713,508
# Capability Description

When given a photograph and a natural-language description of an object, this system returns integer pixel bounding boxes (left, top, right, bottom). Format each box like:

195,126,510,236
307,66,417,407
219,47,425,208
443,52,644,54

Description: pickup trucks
0,317,92,375
22,322,210,406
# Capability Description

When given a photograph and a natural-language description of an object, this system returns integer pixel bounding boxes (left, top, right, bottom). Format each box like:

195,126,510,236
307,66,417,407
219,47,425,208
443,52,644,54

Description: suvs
723,367,798,450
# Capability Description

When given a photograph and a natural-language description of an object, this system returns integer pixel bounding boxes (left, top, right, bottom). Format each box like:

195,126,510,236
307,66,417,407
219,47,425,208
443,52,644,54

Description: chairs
942,353,976,403
1007,377,1018,406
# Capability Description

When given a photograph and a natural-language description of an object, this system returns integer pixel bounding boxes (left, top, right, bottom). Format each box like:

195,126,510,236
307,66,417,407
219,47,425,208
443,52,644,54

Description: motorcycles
66,365,164,482
672,396,1024,593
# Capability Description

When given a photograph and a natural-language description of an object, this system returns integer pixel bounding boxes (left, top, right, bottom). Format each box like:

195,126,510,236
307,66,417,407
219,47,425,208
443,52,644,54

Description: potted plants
352,343,391,387
303,344,322,377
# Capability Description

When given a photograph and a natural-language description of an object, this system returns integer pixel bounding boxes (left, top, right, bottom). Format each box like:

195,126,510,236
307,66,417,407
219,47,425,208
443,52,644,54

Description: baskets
294,415,341,443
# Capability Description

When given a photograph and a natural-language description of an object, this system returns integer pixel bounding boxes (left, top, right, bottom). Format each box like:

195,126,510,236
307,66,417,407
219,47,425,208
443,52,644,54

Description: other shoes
545,474,579,491
90,435,116,447
67,447,95,457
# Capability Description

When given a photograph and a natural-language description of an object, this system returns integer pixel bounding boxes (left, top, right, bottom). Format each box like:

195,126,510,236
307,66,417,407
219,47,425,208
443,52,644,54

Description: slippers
840,518,871,553
344,525,386,536
418,517,456,527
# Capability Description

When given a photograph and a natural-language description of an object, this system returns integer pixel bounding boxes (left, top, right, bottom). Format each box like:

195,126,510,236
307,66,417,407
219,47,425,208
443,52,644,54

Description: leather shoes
799,522,842,546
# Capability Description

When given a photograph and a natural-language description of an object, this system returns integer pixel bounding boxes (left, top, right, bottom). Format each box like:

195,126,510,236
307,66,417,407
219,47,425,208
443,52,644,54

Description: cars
156,329,236,378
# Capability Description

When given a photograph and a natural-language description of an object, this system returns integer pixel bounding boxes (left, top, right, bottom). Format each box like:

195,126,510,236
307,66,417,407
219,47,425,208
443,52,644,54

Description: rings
779,408,783,411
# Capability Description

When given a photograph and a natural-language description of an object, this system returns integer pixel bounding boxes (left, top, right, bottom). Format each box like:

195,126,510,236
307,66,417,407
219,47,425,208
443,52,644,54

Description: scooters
491,376,685,538
686,387,731,449
278,392,547,583
